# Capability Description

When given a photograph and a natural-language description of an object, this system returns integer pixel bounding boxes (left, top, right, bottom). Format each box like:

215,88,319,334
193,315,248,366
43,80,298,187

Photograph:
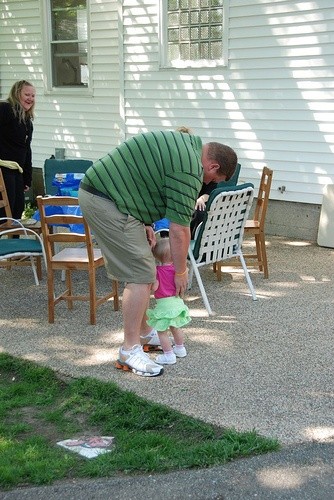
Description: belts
80,183,111,200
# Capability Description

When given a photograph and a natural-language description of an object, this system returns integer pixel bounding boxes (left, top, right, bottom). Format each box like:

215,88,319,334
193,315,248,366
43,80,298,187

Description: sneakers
154,354,176,364
115,344,165,377
139,328,163,351
173,346,187,358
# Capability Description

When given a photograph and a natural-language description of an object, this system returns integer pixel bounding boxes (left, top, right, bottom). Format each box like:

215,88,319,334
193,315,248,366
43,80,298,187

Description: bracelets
175,269,187,276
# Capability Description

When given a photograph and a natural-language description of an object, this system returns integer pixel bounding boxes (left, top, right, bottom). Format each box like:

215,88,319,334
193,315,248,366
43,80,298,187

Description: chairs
0,217,55,299
186,183,257,317
37,196,119,325
43,158,94,256
0,168,54,281
213,166,273,282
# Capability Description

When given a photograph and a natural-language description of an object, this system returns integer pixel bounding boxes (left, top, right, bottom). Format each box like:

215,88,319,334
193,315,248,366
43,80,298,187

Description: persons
146,238,192,364
79,131,238,377
174,127,217,240
0,80,36,260
